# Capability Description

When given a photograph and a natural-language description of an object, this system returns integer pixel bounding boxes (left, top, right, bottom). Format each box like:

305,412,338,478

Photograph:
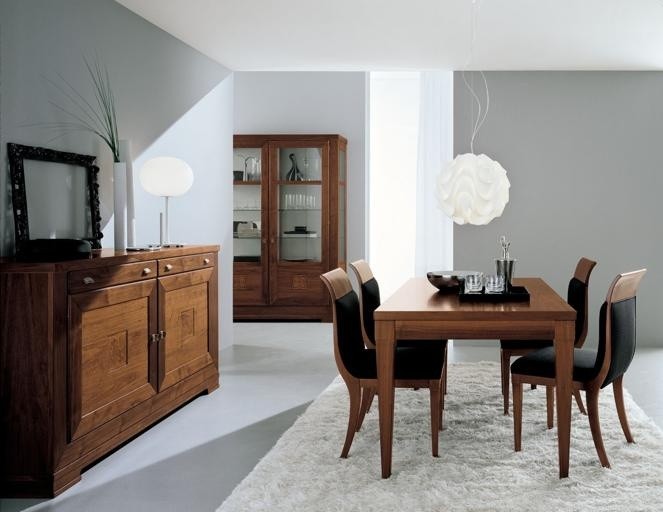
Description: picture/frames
6,142,103,252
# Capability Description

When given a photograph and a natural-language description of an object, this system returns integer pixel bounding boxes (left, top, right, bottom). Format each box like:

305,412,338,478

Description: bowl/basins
425,271,464,295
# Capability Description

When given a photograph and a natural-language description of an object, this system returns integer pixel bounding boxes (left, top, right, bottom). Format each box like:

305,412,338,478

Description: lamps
137,157,193,242
431,0,510,228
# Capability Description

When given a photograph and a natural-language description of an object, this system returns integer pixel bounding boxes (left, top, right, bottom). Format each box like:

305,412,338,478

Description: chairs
320,268,447,459
510,269,649,471
349,260,448,432
499,257,597,416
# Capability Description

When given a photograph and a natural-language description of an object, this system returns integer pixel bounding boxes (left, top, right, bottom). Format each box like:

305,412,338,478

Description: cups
282,193,316,210
484,275,505,293
464,272,482,291
494,259,516,293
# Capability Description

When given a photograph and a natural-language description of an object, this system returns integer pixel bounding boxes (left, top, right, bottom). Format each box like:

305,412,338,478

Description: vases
111,161,127,250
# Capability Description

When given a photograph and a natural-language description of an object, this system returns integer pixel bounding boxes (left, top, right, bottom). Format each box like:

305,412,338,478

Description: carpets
210,361,663,512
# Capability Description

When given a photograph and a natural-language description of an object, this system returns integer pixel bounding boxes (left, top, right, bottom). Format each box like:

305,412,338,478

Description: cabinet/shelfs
0,245,225,499
233,134,347,323
